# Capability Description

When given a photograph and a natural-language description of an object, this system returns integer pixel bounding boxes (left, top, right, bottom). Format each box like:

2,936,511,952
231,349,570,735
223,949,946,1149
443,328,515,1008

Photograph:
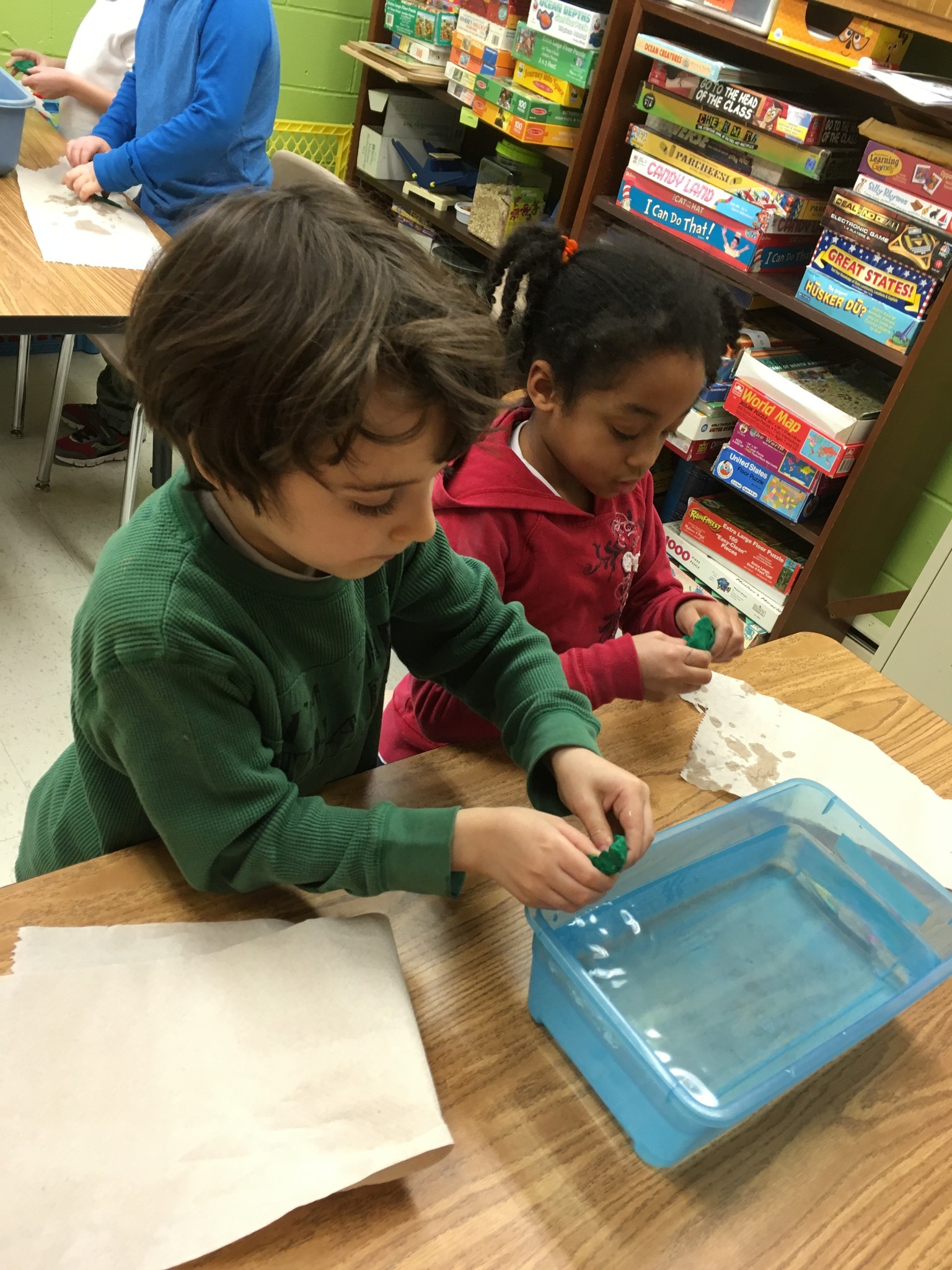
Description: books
632,31,795,93
693,397,736,420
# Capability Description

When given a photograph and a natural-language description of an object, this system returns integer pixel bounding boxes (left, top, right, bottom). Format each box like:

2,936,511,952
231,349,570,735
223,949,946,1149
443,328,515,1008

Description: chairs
31,148,358,527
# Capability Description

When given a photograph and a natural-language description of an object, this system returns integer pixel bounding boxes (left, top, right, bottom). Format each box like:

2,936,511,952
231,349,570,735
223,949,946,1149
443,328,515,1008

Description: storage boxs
384,0,608,150
522,778,952,1170
615,0,952,650
0,63,36,179
391,200,469,257
356,88,466,180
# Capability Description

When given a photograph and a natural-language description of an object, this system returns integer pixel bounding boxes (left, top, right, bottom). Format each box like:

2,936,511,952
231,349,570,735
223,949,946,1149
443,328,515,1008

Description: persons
3,0,151,202
9,180,663,912
370,218,753,780
48,0,290,471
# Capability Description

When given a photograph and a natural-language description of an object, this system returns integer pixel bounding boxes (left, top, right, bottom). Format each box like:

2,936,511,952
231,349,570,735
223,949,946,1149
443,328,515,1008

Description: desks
0,630,952,1270
0,106,179,491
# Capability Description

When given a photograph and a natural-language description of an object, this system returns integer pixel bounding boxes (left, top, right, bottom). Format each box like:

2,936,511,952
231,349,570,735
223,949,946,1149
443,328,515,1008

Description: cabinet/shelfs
558,0,952,657
344,0,638,397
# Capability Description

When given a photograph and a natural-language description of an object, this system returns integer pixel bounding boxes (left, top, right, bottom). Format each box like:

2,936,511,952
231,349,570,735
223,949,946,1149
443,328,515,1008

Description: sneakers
55,423,130,467
62,404,92,430
